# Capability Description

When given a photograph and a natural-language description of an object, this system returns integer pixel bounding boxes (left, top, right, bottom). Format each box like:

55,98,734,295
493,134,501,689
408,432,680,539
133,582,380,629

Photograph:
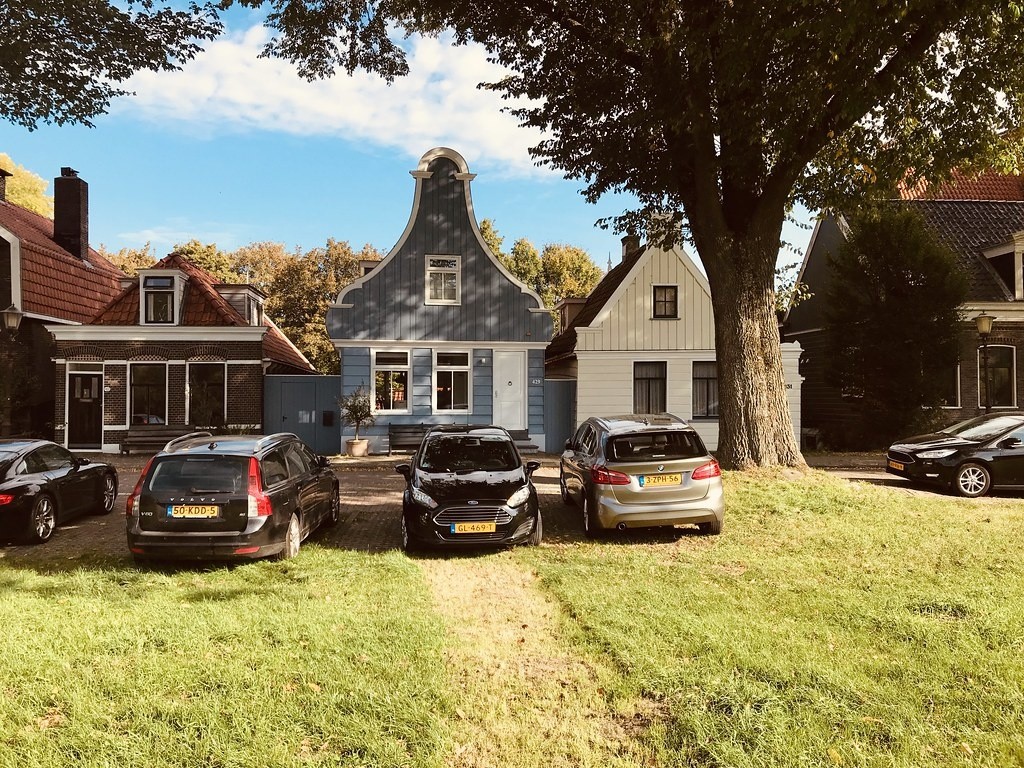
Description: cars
885,412,1024,498
0,437,119,546
394,425,543,556
133,413,165,424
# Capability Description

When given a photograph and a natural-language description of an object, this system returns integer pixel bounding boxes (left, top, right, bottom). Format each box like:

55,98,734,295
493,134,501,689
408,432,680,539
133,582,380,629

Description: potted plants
333,380,375,457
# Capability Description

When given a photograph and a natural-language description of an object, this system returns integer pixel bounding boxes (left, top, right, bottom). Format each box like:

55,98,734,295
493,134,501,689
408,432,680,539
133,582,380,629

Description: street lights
0,302,26,439
971,309,997,414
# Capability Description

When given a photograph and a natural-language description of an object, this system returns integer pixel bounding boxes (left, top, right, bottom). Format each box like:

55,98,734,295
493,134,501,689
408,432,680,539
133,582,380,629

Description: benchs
119,425,195,457
388,423,488,457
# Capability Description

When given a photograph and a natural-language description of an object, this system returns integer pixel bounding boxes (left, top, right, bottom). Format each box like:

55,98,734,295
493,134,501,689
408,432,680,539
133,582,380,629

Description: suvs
126,431,340,573
559,412,724,543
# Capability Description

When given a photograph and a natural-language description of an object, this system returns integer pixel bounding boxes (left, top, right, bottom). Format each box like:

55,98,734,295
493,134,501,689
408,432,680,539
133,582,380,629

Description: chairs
652,434,668,449
615,437,632,451
